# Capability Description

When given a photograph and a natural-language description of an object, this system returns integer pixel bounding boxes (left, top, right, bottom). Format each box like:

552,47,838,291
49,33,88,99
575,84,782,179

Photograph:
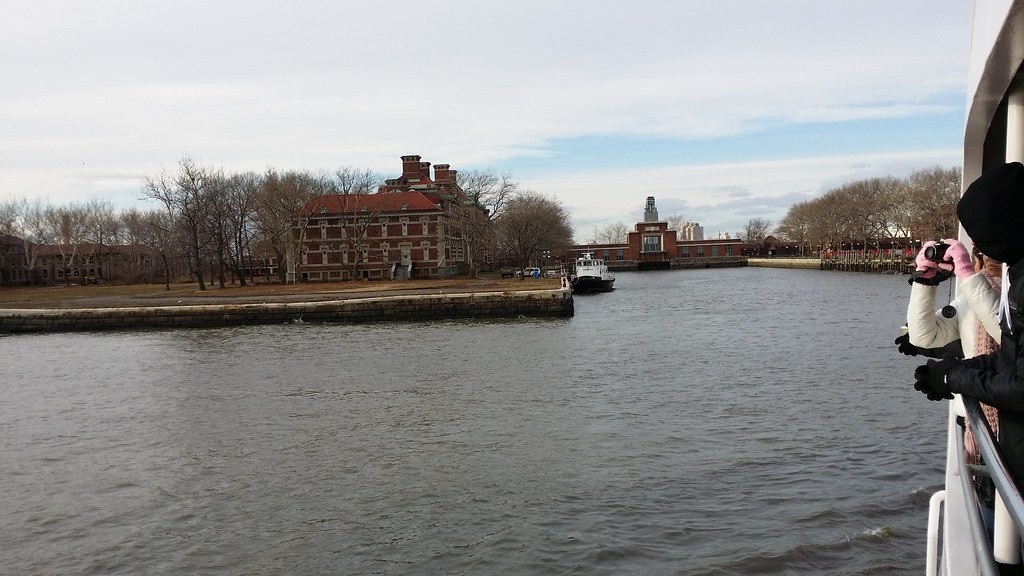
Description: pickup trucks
501,267,514,277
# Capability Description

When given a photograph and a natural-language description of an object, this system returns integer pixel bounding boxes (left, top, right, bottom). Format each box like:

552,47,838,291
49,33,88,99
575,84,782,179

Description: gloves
914,353,964,401
895,323,935,358
939,239,976,281
915,240,938,278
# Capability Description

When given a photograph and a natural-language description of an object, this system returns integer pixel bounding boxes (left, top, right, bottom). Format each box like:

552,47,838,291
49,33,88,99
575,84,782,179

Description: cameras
924,241,954,263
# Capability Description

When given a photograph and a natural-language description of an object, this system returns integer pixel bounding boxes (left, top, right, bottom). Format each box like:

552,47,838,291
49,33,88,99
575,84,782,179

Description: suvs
517,268,540,278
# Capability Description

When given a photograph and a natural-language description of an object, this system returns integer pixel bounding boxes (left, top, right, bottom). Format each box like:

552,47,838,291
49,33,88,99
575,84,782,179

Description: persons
894,162,1024,549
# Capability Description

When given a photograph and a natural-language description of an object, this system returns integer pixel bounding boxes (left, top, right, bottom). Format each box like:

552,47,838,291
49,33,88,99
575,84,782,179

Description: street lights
542,251,550,277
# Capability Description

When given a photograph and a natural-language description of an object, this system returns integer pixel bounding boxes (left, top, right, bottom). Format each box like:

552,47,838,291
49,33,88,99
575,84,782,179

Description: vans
547,271,558,276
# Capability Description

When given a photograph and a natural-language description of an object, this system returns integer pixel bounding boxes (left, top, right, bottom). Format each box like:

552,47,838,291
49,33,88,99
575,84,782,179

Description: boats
569,254,616,293
